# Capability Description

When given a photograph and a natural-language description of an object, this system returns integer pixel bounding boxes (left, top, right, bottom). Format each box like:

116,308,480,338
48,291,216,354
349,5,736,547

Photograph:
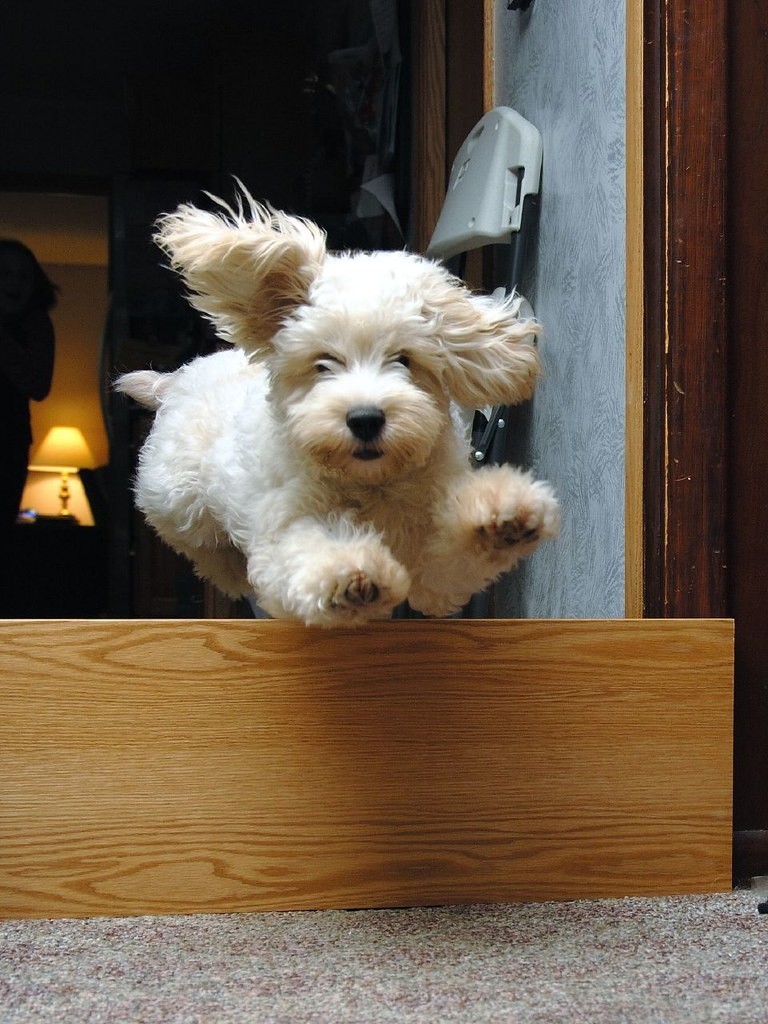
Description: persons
0,238,57,542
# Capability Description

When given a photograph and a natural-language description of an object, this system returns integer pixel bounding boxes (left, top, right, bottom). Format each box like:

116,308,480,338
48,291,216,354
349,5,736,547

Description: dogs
111,173,563,631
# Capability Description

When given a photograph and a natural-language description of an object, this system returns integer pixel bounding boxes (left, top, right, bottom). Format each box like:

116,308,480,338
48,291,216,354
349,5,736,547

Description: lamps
27,424,95,516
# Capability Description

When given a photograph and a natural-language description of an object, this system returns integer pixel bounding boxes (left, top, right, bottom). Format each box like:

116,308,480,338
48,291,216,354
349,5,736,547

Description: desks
6,522,104,620
